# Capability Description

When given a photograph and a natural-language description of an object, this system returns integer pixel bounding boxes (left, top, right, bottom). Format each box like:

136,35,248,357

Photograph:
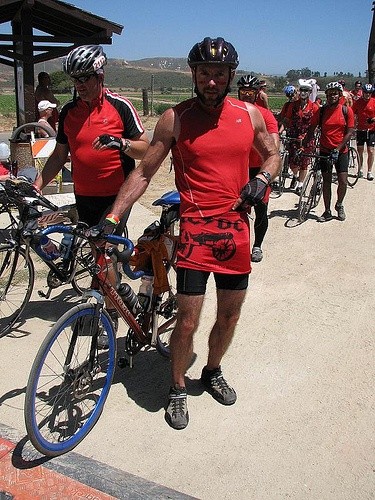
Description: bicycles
0,176,95,338
332,128,369,185
275,135,302,195
291,149,333,223
24,190,182,457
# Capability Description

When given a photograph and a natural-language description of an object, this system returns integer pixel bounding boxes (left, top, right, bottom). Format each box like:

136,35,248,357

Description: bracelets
106,215,121,225
32,183,40,192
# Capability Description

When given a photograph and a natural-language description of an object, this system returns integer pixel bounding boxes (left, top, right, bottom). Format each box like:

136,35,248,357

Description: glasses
71,75,94,83
239,90,255,95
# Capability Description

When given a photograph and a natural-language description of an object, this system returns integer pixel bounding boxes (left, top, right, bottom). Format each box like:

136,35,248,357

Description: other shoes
290,175,302,192
367,173,374,180
251,247,263,262
281,172,294,179
97,308,118,350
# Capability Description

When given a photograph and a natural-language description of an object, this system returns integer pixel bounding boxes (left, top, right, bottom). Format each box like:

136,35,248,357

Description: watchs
258,171,271,184
122,139,131,152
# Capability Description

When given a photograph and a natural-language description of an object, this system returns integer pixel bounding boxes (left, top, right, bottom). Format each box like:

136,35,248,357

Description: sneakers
165,382,189,430
335,203,346,221
317,211,333,222
201,365,237,406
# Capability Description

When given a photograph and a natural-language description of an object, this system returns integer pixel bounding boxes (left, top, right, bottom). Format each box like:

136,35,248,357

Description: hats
38,100,57,111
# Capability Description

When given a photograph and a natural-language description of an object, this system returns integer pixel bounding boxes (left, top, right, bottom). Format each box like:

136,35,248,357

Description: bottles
40,235,61,261
59,231,74,258
137,265,155,312
318,170,322,179
116,284,144,317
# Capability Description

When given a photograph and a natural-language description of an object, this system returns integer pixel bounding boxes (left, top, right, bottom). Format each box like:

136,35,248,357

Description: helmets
237,75,259,89
187,37,240,69
363,84,375,93
325,82,343,94
284,86,296,93
63,45,107,77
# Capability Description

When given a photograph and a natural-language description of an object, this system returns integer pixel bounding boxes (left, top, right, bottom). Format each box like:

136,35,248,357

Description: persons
35,99,57,137
34,71,61,131
236,74,375,262
28,43,150,349
89,37,283,430
